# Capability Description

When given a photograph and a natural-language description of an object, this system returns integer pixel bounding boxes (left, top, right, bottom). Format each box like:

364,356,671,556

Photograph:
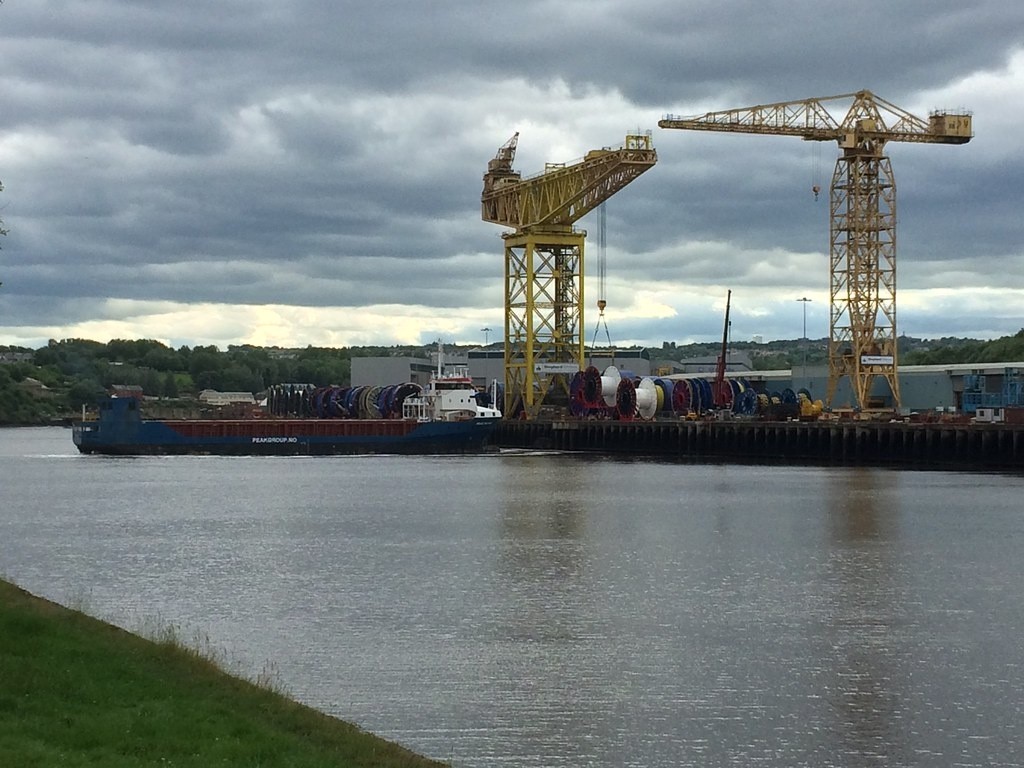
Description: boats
71,391,499,452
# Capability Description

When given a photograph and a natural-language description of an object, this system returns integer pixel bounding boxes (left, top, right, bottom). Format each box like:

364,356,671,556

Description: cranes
482,129,658,415
657,87,974,414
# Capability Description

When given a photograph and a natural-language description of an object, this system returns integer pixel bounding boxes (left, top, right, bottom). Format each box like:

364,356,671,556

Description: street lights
796,297,812,368
